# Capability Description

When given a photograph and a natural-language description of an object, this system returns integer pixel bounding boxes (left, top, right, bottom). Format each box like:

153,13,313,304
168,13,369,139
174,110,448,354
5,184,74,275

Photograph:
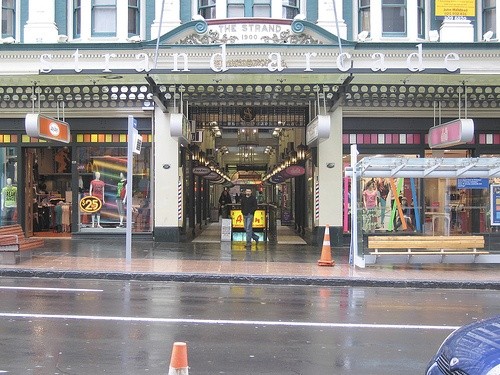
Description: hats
402,197,408,201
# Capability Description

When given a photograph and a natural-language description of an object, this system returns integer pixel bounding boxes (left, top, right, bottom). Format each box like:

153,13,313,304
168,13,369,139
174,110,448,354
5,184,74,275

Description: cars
426,313,500,375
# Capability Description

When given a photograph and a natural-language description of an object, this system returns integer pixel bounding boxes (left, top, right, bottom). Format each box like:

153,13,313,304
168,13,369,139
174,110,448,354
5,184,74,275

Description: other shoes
97,225,103,229
245,243,251,247
256,236,259,242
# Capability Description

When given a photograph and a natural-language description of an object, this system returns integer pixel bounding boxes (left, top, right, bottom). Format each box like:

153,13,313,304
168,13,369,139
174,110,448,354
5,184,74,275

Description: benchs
0,224,44,264
368,236,489,264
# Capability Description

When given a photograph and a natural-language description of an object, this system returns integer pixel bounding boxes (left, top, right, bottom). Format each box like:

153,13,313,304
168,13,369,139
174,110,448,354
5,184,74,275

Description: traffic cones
167,341,188,375
316,223,336,267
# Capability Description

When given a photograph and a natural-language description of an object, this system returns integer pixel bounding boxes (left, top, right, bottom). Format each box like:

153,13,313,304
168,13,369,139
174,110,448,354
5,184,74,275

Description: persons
1,178,17,225
219,190,231,218
363,180,414,233
115,172,127,227
235,186,259,247
90,172,106,228
348,184,351,230
